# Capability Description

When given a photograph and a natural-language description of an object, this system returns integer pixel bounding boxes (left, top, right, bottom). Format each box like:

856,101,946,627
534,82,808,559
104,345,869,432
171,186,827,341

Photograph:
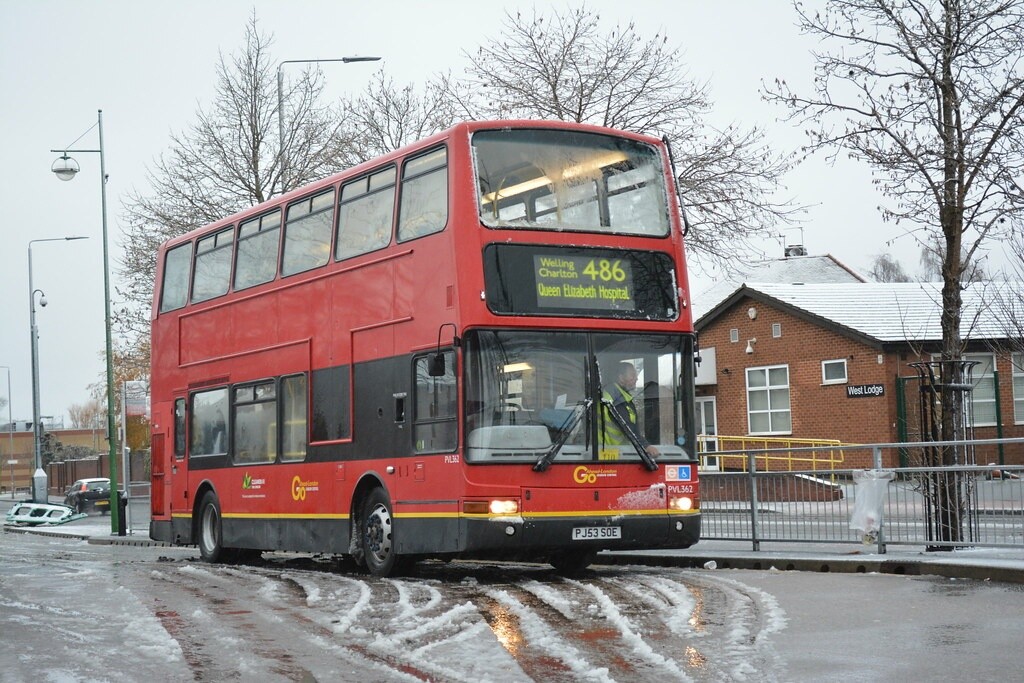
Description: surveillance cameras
40,296,47,307
746,347,754,355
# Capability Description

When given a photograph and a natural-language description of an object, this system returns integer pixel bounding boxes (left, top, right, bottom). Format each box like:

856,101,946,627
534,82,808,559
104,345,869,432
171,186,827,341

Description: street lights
276,55,383,200
49,110,121,537
26,236,92,505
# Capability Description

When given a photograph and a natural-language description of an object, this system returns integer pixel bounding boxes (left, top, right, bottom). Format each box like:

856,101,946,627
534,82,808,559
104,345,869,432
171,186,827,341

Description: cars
61,476,126,519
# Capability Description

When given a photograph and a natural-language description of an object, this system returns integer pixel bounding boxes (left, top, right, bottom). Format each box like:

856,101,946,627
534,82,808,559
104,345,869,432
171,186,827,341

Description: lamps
721,369,731,374
746,337,756,354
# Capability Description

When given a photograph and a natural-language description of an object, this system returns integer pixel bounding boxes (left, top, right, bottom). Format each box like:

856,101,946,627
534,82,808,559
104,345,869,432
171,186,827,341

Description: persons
597,362,662,457
177,403,228,455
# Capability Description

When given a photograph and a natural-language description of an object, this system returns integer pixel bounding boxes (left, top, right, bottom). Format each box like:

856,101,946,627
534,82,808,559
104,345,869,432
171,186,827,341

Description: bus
147,121,704,576
